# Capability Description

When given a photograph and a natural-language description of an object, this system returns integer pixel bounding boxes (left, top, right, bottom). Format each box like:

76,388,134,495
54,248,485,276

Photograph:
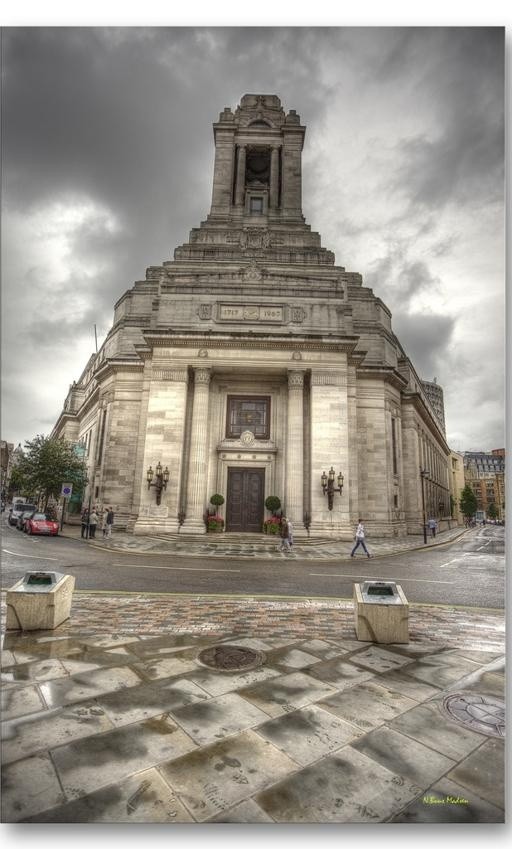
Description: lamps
143,460,169,506
320,463,344,512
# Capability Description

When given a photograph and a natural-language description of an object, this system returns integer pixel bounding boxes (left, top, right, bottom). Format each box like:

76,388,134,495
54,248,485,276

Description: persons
279,518,291,553
351,518,375,560
284,517,293,549
81,505,114,539
428,517,436,538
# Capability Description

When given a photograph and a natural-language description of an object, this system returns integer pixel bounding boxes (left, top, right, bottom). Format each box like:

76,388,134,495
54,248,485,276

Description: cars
9,503,60,537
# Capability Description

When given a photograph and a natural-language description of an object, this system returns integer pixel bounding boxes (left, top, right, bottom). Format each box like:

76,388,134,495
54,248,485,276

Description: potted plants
262,493,287,535
207,493,228,532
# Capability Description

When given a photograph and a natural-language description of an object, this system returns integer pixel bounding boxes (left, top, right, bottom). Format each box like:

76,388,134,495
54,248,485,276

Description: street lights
420,468,430,543
